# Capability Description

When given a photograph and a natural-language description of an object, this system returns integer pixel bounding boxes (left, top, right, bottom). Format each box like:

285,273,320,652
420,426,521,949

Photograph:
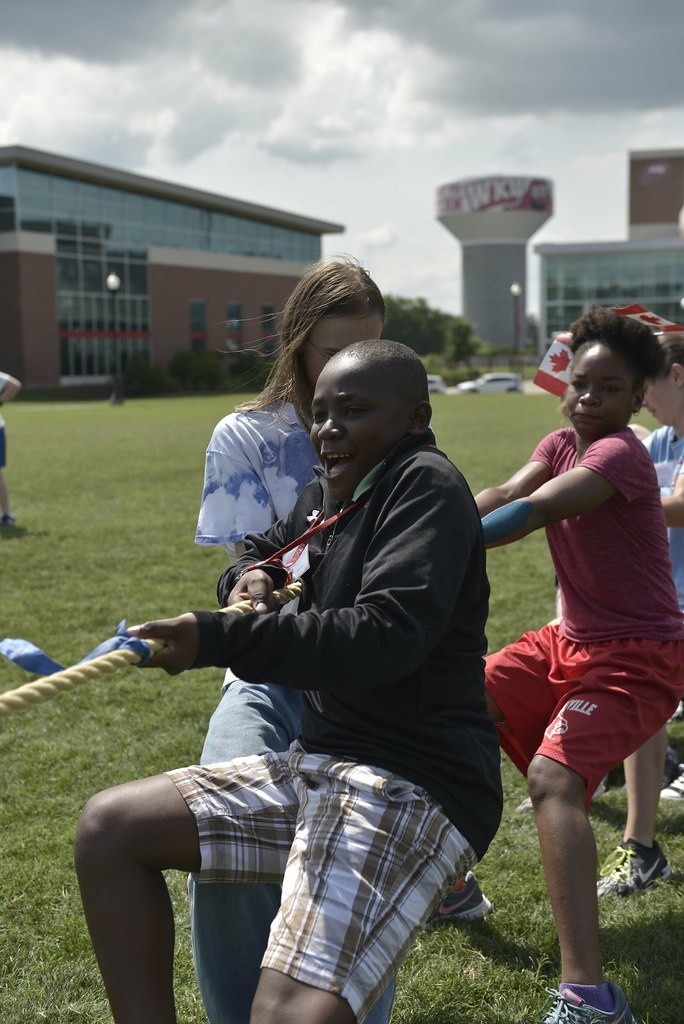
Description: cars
428,374,444,391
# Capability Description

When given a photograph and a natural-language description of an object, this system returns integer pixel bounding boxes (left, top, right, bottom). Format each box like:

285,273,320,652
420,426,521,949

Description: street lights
511,283,522,357
106,272,127,403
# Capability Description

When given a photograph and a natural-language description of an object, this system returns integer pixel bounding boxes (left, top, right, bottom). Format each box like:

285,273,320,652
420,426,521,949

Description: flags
610,304,683,332
533,333,574,398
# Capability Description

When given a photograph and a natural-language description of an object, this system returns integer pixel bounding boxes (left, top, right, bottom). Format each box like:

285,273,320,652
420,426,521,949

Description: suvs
457,372,523,393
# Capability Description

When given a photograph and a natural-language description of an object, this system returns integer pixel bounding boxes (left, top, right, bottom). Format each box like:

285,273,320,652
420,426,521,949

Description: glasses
307,334,337,363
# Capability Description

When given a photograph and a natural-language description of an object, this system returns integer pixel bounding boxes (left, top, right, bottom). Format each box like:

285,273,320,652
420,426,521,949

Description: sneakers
519,774,606,807
659,763,684,802
597,838,670,899
0,513,15,525
420,872,492,923
538,979,637,1024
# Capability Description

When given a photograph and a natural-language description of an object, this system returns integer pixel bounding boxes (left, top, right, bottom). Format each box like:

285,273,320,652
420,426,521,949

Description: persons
186,251,392,1023
0,371,22,528
474,303,684,1023
75,341,504,1023
